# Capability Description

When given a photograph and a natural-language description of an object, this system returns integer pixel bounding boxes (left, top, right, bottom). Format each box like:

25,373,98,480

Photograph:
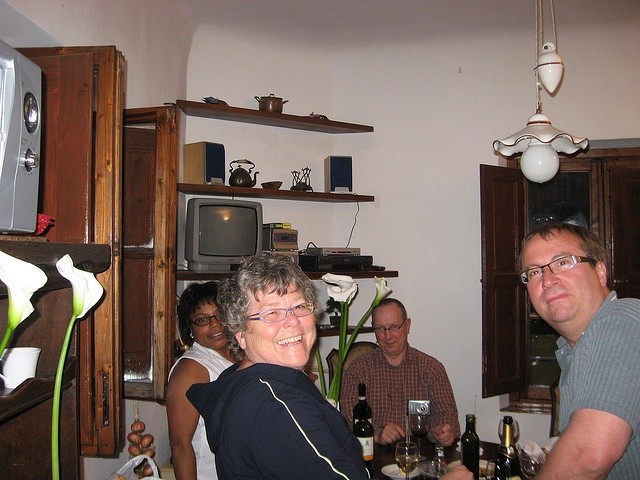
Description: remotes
371,266,386,271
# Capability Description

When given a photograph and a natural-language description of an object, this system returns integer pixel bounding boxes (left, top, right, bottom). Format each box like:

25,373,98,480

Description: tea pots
229,159,259,187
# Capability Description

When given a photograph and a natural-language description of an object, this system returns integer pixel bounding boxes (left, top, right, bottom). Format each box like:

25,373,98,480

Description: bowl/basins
261,181,282,190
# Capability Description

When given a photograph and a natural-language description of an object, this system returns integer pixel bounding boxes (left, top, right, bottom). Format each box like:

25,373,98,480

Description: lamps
536,42,565,95
492,109,592,186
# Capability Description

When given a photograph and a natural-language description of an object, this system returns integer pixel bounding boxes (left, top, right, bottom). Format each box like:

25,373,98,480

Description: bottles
430,444,449,480
353,402,374,471
494,416,523,480
461,414,479,480
352,383,375,471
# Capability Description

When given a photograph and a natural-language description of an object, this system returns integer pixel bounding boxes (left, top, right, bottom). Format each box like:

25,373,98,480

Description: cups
0,346,42,390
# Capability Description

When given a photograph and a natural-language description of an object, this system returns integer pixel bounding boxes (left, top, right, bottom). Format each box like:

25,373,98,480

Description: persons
186,252,371,479
517,223,640,480
166,283,236,480
341,300,461,447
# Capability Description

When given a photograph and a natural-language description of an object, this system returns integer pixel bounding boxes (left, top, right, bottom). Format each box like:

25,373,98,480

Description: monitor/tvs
185,197,263,274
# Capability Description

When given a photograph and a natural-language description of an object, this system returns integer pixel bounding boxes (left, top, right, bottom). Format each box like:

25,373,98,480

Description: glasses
245,301,315,323
372,318,406,333
517,254,597,283
189,313,223,327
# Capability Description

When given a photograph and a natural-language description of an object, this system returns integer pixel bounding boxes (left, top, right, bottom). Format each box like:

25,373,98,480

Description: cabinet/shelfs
168,99,400,338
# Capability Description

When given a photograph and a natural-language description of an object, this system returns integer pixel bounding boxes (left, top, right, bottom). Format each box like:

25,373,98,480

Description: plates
448,459,495,480
381,463,420,480
419,460,448,477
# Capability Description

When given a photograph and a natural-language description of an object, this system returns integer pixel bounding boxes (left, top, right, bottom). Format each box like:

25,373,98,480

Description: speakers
182,140,226,185
324,155,354,193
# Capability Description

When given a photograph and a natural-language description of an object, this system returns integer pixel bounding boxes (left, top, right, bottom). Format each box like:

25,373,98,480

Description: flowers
322,273,394,406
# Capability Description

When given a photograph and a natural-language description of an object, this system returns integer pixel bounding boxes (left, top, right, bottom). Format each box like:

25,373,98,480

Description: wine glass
410,414,430,462
521,456,540,480
498,419,519,444
395,442,420,480
485,459,495,480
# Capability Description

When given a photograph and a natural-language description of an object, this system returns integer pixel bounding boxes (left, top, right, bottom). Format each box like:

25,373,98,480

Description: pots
254,93,289,114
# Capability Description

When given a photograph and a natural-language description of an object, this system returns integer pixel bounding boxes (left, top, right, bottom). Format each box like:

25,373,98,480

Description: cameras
407,399,432,416
